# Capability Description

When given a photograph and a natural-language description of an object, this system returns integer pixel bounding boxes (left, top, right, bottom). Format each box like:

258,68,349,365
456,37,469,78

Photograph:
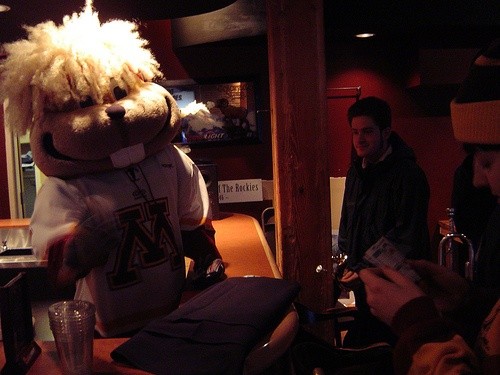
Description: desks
1,208,299,375
174,129,257,150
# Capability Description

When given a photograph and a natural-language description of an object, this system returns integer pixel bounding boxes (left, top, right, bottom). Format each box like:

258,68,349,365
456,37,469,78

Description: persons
449,137,497,278
338,91,437,311
358,31,500,375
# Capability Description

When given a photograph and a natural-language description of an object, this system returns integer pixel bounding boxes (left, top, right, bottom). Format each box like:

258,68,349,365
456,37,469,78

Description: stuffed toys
2,0,225,340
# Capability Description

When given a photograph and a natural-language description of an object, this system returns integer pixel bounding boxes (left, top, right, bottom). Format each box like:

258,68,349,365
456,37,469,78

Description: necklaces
126,163,162,214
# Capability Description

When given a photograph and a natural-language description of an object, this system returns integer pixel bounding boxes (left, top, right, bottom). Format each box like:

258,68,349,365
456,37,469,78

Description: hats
450,42,500,146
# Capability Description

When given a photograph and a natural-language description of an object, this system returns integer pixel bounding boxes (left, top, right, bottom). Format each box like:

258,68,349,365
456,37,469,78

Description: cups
49,300,96,374
